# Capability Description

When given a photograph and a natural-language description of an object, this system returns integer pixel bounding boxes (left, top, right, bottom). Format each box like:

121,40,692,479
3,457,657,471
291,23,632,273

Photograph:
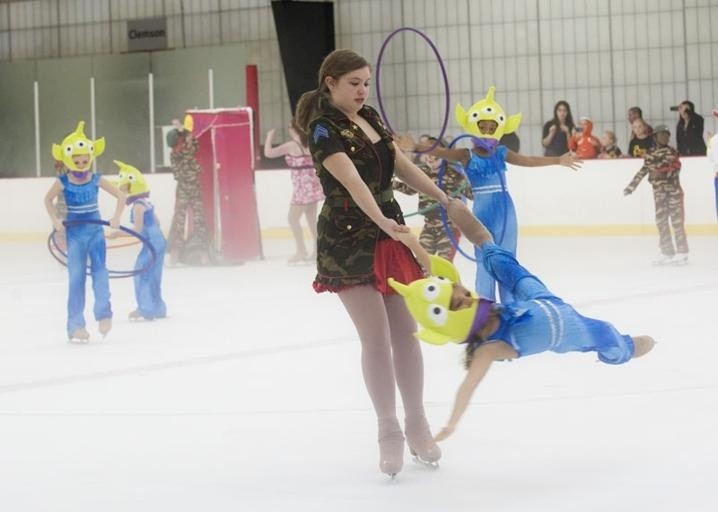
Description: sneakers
378,421,404,473
408,414,440,462
652,253,689,264
289,249,319,263
631,336,654,356
73,309,143,339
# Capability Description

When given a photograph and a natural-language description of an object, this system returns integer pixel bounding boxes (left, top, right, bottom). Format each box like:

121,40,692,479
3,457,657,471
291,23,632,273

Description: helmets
652,125,671,135
428,136,450,147
167,129,181,146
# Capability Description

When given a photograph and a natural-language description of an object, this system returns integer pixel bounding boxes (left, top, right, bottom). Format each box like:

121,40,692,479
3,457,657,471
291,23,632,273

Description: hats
52,121,104,180
111,160,149,204
387,255,494,344
455,87,522,149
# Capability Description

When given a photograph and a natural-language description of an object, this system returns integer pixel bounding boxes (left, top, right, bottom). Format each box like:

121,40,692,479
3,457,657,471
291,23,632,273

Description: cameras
574,126,584,133
669,106,678,111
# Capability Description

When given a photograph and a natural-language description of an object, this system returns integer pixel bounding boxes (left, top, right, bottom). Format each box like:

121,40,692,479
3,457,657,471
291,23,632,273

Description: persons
44,123,125,343
712,110,718,212
266,116,323,261
163,130,208,266
622,125,689,268
396,87,584,295
392,136,471,261
412,100,707,158
295,49,451,478
108,165,164,322
397,198,656,442
